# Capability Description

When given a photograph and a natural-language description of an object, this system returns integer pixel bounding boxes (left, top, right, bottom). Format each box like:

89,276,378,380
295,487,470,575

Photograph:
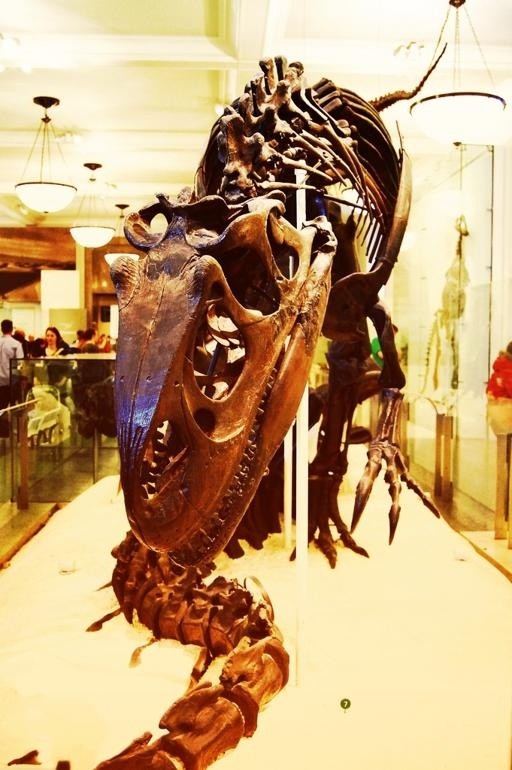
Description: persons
1,316,120,441
371,322,403,373
486,341,512,401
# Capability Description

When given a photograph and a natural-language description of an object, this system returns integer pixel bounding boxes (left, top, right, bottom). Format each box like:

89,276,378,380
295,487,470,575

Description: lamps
14,94,140,270
407,1,506,141
